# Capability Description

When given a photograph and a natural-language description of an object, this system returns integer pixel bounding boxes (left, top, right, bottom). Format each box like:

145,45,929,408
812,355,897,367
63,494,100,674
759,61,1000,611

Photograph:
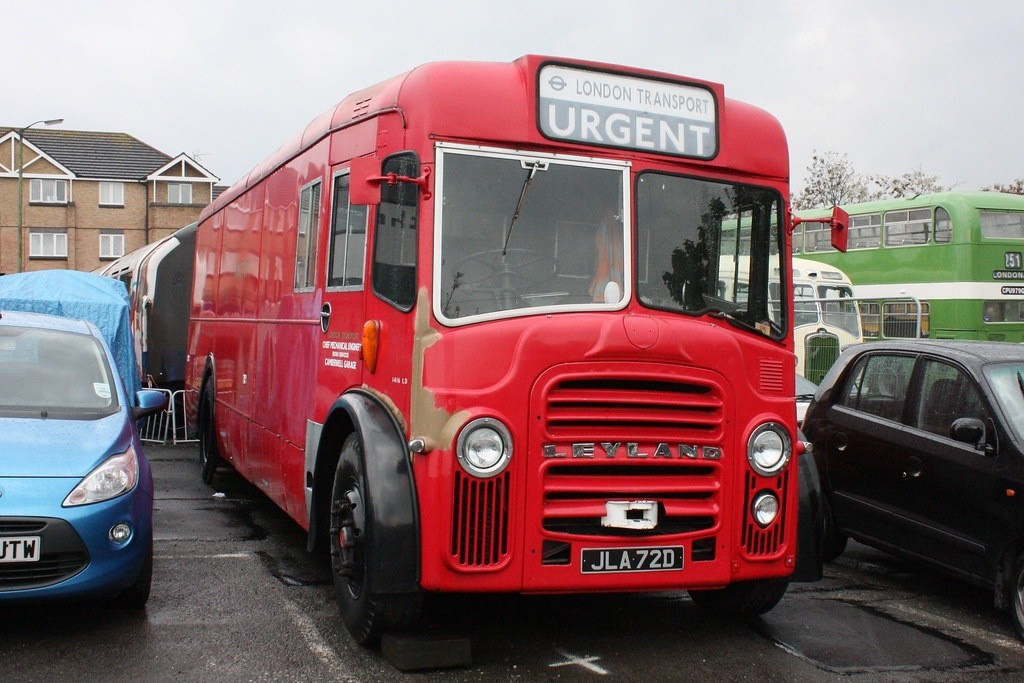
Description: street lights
18,116,63,273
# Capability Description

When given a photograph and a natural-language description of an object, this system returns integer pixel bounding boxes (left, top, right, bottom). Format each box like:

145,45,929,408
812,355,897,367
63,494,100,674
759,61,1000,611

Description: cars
795,372,819,430
248,290,323,431
0,309,168,607
802,339,1024,633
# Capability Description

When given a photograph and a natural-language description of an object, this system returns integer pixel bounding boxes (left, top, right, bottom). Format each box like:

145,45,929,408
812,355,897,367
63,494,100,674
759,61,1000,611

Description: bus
87,222,198,399
719,192,1024,399
718,254,865,397
186,56,849,647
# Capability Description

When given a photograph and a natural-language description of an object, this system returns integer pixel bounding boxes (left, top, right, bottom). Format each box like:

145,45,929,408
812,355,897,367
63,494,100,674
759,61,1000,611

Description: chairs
729,237,930,253
927,379,962,416
